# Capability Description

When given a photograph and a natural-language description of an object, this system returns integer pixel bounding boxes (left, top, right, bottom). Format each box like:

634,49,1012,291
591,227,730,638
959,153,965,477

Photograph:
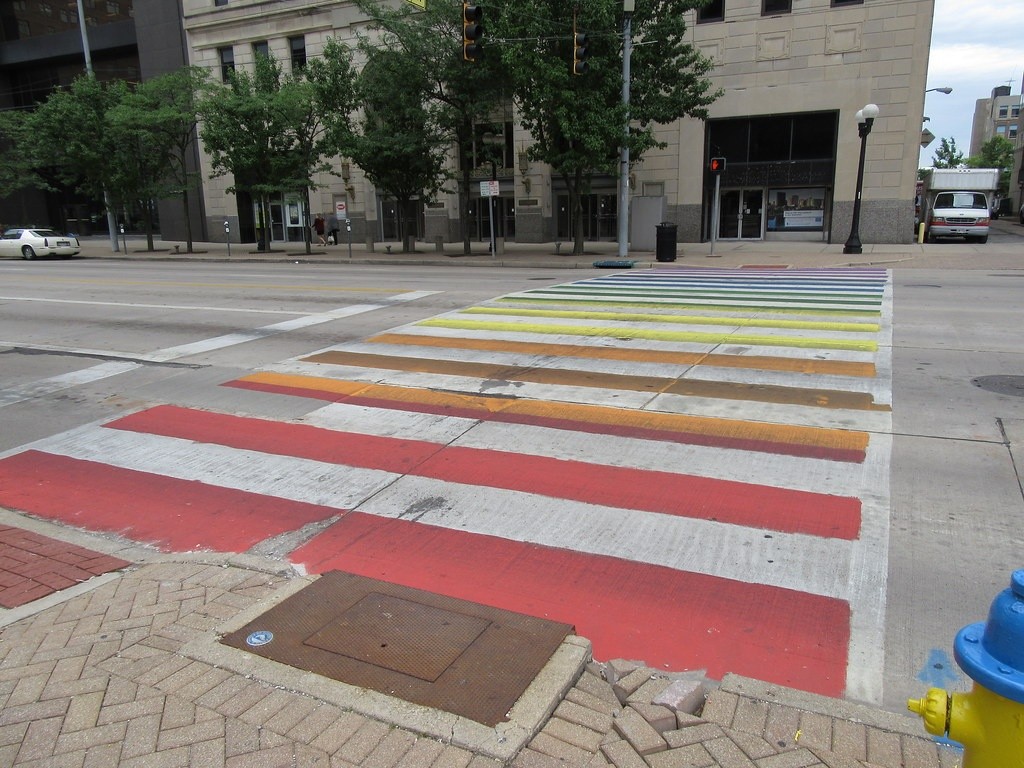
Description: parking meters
224,219,231,256
344,219,353,258
119,224,128,255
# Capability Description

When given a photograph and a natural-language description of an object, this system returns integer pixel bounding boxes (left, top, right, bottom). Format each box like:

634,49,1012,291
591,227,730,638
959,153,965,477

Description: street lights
842,102,880,255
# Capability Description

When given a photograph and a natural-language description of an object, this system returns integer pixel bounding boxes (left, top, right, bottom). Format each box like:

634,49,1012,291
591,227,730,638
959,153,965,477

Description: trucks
919,168,1000,244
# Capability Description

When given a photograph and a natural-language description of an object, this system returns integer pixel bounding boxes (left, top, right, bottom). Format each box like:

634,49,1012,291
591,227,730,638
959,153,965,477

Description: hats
327,209,333,214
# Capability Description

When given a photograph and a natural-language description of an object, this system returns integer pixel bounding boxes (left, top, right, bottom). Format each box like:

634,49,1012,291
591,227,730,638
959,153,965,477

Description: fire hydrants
903,569,1023,768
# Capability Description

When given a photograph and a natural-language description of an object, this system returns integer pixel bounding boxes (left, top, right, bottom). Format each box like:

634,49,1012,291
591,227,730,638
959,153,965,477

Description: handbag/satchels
332,222,340,231
326,231,335,242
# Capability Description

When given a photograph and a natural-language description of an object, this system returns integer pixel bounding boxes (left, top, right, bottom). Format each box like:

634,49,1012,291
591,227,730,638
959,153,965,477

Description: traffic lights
573,31,590,77
463,3,485,62
709,157,727,172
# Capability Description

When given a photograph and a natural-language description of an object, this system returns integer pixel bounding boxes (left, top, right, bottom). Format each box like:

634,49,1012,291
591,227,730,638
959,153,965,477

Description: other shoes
317,245,321,246
334,243,338,245
324,242,326,247
328,243,332,245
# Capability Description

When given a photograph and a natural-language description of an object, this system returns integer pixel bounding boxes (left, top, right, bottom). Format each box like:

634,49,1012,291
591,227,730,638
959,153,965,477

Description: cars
1019,204,1024,224
0,228,81,261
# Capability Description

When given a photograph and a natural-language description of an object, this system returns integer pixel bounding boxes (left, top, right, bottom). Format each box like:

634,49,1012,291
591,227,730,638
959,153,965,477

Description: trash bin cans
654,221,678,262
515,197,546,242
424,198,450,243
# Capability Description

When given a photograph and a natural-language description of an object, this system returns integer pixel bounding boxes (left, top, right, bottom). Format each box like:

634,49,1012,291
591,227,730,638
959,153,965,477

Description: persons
310,213,326,246
326,211,340,245
